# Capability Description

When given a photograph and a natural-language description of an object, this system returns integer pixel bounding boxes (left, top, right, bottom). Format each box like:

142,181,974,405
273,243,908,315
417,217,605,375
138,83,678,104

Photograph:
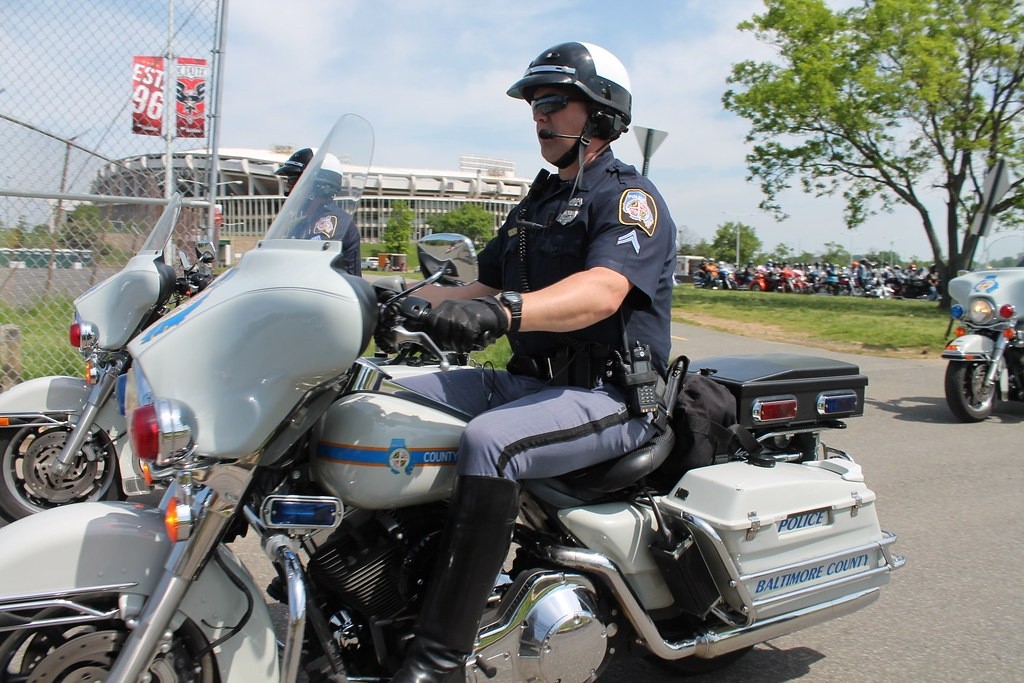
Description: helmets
699,255,924,274
506,42,633,126
273,147,343,191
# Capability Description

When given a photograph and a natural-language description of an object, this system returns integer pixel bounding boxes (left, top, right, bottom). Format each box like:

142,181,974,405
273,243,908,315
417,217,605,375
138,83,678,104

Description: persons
274,148,361,276
699,258,941,302
374,41,677,683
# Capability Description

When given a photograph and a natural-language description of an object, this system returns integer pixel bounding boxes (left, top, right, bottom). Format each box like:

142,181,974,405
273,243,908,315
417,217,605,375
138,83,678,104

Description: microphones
538,129,599,139
284,191,289,196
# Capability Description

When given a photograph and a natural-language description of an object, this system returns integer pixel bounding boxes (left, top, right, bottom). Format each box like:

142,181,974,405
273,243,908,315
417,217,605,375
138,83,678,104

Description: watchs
501,291,522,333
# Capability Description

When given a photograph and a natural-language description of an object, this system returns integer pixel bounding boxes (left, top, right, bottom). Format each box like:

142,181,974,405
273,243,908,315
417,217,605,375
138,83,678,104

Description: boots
389,473,518,683
267,577,288,607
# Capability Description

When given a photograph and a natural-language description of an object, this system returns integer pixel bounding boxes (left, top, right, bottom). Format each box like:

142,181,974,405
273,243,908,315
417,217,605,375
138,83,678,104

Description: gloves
189,273,213,298
423,295,508,355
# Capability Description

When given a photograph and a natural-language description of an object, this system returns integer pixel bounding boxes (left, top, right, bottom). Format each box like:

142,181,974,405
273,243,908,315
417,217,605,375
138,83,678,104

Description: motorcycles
0,112,909,682
939,234,1024,422
692,258,940,302
0,190,228,523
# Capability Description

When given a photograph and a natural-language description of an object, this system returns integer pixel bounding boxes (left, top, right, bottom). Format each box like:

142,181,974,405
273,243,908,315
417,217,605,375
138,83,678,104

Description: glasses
531,94,585,115
287,176,297,184
